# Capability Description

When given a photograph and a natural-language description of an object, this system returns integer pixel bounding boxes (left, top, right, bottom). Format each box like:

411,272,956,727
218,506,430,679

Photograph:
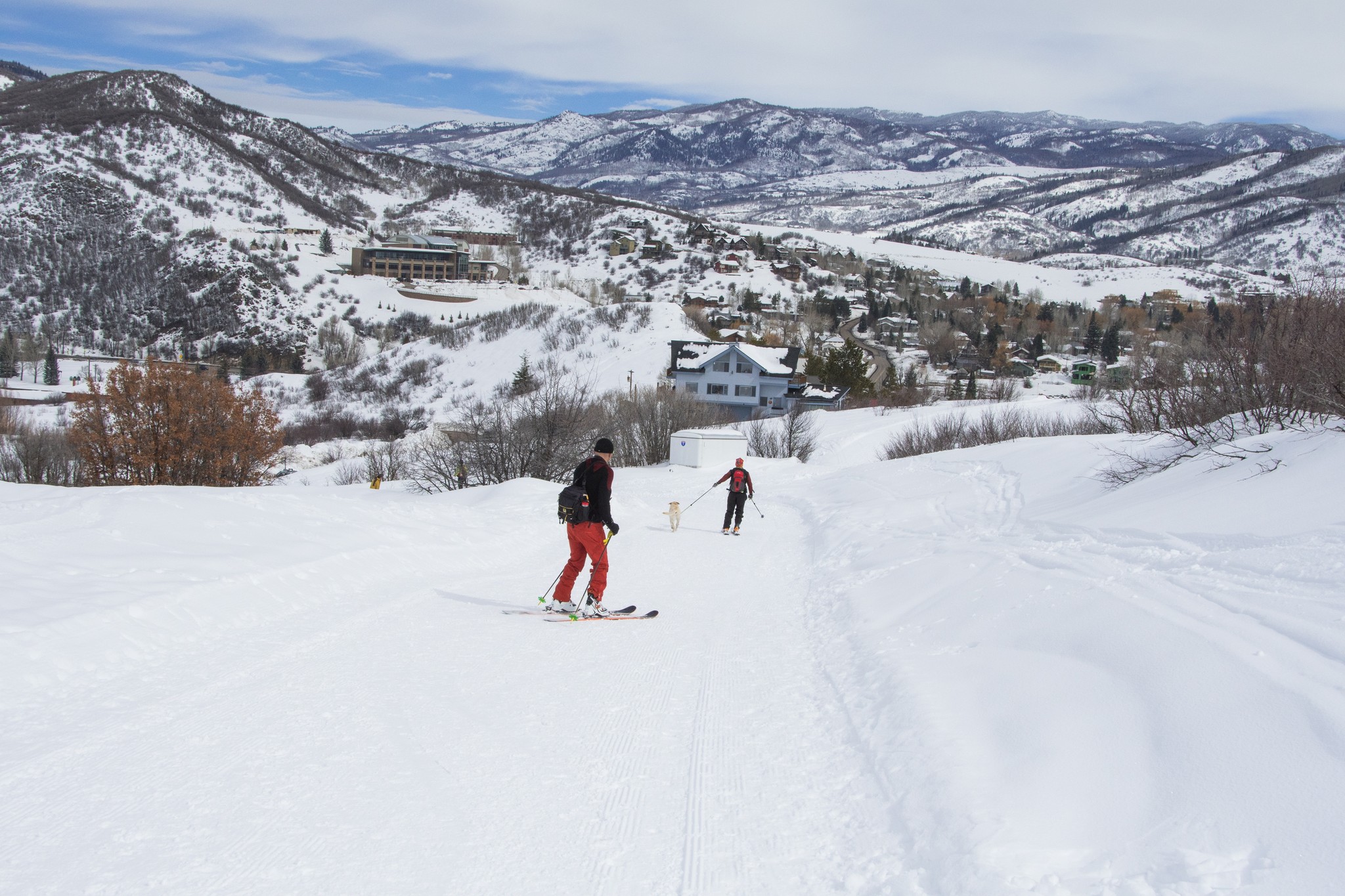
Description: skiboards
502,605,659,622
722,527,740,536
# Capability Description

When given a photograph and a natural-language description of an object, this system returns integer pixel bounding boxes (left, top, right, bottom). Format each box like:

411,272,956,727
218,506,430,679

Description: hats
736,458,743,467
594,438,614,454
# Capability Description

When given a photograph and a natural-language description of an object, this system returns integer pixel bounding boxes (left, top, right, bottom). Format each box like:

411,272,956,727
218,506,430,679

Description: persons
84,348,86,356
453,460,469,489
550,437,619,621
67,348,69,355
370,474,384,489
713,459,754,536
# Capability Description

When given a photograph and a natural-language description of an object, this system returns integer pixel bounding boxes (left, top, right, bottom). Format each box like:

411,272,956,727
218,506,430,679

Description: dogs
661,501,682,533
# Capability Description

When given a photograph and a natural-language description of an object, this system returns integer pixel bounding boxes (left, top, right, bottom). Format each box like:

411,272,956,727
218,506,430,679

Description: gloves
605,518,619,536
713,482,719,488
748,494,753,500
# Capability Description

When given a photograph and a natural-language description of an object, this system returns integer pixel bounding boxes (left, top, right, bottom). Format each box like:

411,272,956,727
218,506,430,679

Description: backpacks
557,461,591,525
730,470,745,492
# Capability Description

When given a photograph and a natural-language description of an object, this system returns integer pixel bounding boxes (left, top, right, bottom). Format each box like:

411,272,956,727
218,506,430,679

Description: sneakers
585,591,608,617
552,596,577,612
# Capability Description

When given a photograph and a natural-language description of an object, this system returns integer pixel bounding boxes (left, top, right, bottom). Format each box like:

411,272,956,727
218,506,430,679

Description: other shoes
733,525,739,532
723,528,728,533
